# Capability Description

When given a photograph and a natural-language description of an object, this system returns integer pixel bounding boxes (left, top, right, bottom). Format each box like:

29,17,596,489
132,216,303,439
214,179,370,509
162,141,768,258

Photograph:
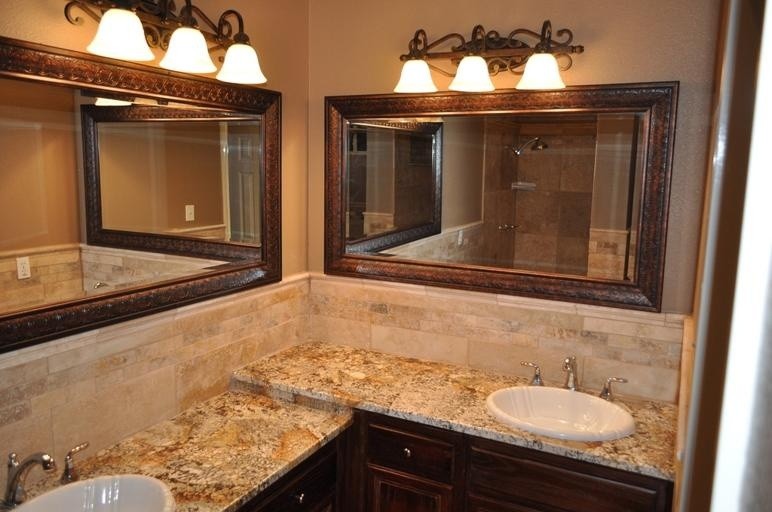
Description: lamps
64,1,267,87
393,19,584,95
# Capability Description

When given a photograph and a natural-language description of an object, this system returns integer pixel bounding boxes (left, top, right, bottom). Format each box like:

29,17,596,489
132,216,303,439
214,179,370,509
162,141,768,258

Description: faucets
93,282,109,288
562,355,579,392
5,451,57,505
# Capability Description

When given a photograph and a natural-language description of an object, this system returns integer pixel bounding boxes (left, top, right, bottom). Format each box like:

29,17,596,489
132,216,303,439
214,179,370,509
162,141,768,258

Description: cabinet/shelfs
234,428,352,512
354,408,672,512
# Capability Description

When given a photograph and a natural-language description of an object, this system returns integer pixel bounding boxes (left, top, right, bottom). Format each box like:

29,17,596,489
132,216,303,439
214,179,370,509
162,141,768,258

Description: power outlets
16,257,32,280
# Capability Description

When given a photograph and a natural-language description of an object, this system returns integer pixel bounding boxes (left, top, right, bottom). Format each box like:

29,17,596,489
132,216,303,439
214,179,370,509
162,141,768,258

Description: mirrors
77,100,263,261
323,81,679,312
2,37,280,356
345,118,441,253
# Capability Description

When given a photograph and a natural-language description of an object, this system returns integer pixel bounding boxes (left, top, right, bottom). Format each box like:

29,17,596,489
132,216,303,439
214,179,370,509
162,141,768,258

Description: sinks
8,473,176,512
487,385,636,442
